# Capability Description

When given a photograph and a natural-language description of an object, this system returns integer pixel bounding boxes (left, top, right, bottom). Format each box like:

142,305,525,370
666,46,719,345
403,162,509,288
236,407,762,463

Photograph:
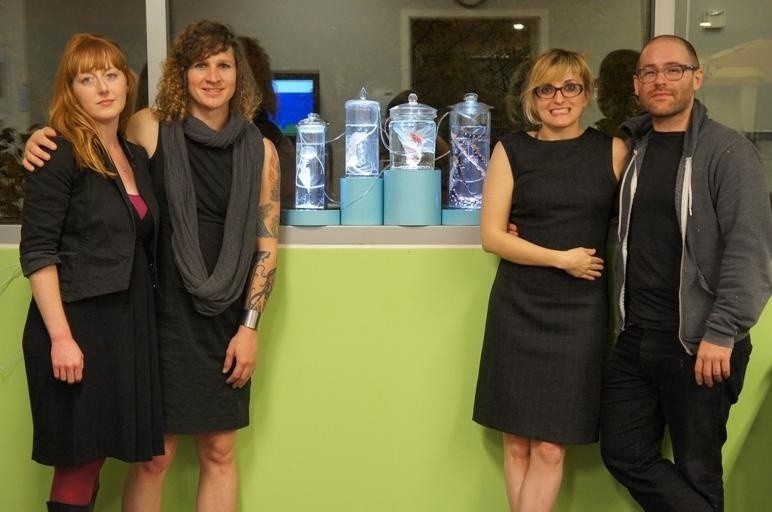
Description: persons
378,88,450,194
22,18,281,512
473,48,634,512
16,31,166,512
506,35,771,512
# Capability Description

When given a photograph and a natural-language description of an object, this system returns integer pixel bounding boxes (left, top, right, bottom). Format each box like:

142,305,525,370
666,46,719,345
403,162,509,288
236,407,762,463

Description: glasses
636,63,698,83
535,84,584,99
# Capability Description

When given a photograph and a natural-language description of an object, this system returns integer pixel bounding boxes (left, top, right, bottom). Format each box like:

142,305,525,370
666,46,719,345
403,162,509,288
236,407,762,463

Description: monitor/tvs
267,69,321,144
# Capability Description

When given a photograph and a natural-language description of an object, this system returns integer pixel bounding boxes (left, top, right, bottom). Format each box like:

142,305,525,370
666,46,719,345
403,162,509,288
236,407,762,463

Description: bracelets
241,309,261,331
